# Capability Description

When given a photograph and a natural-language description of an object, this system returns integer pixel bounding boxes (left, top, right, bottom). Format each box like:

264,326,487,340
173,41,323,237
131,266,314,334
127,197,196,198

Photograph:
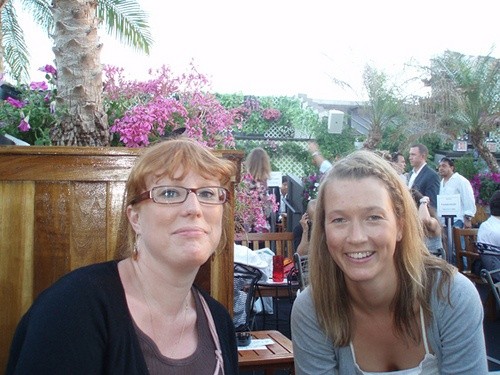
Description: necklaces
167,300,189,340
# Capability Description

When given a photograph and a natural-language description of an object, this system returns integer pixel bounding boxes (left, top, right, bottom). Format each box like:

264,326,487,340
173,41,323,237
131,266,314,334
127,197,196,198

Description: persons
291,149,490,375
392,142,500,259
244,142,332,256
5,136,239,375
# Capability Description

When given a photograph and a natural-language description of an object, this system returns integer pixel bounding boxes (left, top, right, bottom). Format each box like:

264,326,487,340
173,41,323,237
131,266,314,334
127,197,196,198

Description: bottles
235,324,252,347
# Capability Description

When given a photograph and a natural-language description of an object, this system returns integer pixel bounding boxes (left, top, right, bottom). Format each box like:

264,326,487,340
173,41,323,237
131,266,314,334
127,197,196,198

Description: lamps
328,109,344,135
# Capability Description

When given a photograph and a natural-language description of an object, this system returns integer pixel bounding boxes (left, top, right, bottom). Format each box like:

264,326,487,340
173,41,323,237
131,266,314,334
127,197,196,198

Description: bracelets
419,201,427,204
466,216,472,221
308,151,322,159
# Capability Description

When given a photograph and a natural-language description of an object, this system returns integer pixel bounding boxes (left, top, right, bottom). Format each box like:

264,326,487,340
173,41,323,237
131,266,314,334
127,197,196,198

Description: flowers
303,172,322,208
470,173,500,207
0,63,285,150
232,173,278,234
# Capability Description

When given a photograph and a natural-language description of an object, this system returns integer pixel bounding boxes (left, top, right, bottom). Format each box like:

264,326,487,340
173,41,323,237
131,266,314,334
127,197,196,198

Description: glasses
127,185,230,206
393,152,397,158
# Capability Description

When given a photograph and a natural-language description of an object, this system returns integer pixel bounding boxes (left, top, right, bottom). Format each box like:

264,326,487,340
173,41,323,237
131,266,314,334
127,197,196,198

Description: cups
273,254,284,282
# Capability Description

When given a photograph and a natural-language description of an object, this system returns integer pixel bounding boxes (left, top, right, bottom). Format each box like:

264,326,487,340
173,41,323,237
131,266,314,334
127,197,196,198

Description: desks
243,279,295,297
237,330,297,375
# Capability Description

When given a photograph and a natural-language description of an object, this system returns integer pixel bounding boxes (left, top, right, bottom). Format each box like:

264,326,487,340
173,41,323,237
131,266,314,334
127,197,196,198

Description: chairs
453,214,500,305
297,250,312,302
234,232,295,258
233,261,266,333
